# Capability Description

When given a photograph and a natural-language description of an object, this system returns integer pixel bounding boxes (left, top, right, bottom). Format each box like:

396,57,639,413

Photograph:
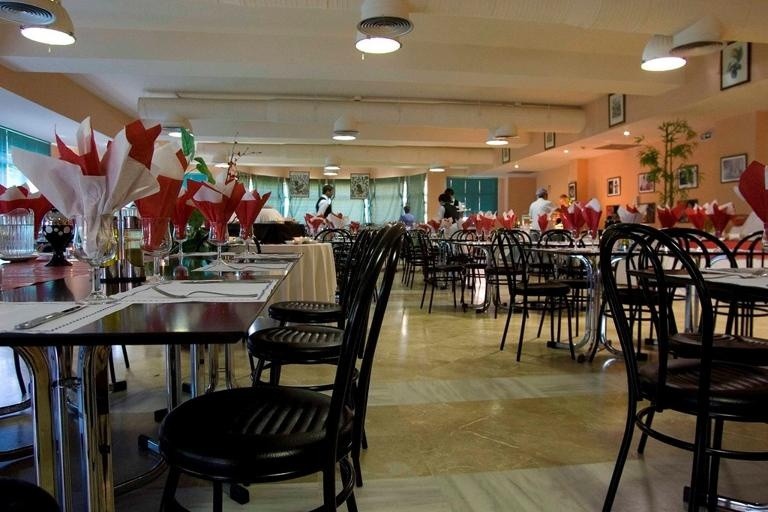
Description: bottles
98,215,146,284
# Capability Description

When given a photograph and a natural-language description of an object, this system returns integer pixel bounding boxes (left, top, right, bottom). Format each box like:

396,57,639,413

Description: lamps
352,28,402,54
20,0,76,45
638,32,687,72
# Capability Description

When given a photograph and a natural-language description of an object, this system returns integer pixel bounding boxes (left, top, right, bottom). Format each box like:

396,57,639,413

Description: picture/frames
567,181,577,201
500,147,510,164
605,93,626,127
604,153,748,197
720,41,751,91
542,131,555,150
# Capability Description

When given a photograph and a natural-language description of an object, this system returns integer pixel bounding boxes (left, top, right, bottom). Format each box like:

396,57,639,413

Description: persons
528,188,554,233
399,206,414,225
316,185,342,218
438,194,457,222
553,194,570,225
443,188,465,219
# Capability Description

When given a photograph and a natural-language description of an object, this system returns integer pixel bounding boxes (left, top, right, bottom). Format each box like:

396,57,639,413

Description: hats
535,188,548,196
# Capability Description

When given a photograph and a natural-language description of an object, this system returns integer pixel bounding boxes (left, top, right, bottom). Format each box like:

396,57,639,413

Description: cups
0,208,34,255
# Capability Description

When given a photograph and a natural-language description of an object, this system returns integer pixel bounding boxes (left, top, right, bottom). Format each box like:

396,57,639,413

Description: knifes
14,304,86,331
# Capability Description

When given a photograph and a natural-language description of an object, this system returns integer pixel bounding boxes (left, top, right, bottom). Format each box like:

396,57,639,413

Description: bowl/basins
285,236,319,246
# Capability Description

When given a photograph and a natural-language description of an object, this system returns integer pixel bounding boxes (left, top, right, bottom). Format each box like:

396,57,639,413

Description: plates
0,255,40,261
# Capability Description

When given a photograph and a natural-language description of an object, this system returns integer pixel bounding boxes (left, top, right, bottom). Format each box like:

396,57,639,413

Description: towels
11,118,273,240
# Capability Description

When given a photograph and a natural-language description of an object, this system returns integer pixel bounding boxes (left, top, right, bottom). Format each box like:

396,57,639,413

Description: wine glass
208,222,231,266
409,220,768,281
239,223,254,256
138,216,173,285
173,223,189,256
69,213,119,305
173,257,189,281
240,259,256,282
